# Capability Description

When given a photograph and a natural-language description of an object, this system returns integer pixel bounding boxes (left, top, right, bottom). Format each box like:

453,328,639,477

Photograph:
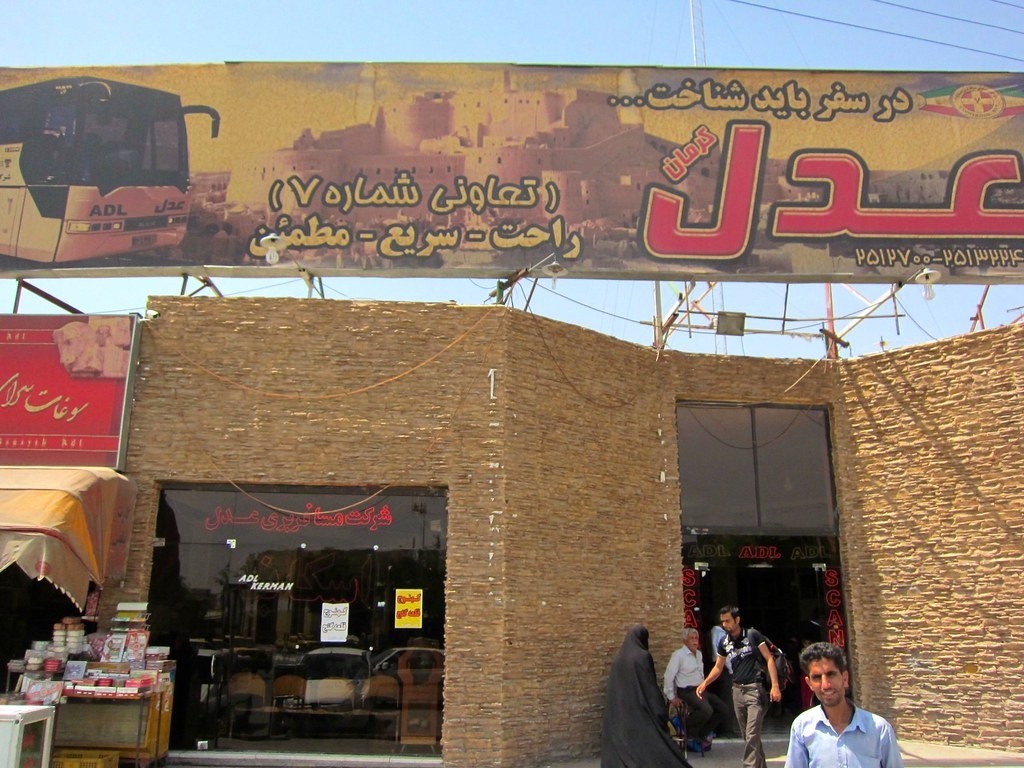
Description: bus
0,76,224,267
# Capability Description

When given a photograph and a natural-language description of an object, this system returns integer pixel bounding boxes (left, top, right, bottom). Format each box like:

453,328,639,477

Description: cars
185,632,446,728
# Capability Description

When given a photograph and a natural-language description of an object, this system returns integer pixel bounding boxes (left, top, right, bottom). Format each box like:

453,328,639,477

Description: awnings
0,465,138,613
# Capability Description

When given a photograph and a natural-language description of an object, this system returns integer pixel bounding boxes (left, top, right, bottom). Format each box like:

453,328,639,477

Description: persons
601,626,691,768
697,606,782,768
785,637,818,720
782,642,904,768
663,628,728,750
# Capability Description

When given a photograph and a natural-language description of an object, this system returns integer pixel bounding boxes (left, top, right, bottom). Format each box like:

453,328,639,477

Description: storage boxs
51,749,120,768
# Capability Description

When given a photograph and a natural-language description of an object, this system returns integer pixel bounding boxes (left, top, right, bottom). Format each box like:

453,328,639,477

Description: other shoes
785,710,794,717
772,711,782,718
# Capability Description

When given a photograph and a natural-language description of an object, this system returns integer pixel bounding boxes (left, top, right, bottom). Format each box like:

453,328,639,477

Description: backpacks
747,628,792,693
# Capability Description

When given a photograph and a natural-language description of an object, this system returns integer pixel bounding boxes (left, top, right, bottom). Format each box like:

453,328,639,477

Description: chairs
306,678,355,729
356,674,399,728
271,676,306,734
225,656,272,740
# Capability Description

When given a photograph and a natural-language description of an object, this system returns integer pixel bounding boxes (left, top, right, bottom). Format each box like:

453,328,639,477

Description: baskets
52,749,120,768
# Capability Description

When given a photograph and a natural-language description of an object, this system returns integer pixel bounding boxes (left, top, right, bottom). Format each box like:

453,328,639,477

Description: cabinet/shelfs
396,650,442,748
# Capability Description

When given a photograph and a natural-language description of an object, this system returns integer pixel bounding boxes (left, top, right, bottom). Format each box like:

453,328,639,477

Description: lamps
501,251,567,296
261,234,311,286
836,265,939,338
